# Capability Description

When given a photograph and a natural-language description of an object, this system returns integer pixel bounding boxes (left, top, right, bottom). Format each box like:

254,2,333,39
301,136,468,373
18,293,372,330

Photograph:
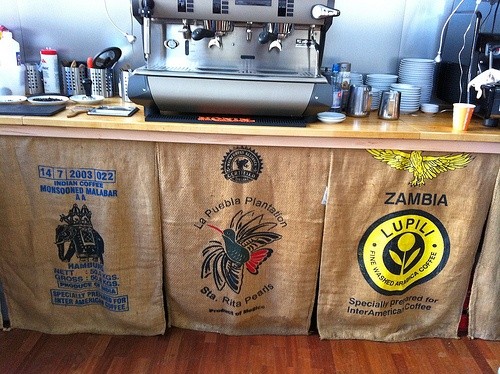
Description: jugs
379,90,401,120
346,84,372,117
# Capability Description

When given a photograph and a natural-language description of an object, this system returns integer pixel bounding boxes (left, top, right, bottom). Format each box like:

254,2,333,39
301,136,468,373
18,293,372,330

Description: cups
241,55,256,73
40,50,61,95
452,103,476,131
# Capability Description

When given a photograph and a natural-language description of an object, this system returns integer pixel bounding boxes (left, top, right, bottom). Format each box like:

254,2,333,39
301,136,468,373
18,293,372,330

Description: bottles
332,62,350,114
0,31,25,96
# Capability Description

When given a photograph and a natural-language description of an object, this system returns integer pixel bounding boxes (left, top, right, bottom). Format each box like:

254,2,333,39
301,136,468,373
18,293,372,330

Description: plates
29,95,69,105
69,95,105,104
318,112,346,123
0,96,28,104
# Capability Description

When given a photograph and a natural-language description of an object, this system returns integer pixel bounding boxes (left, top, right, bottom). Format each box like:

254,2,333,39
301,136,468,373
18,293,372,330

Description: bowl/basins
337,58,438,114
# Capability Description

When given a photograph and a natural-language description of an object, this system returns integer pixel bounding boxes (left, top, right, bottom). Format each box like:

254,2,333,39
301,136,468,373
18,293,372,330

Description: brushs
66,105,95,118
